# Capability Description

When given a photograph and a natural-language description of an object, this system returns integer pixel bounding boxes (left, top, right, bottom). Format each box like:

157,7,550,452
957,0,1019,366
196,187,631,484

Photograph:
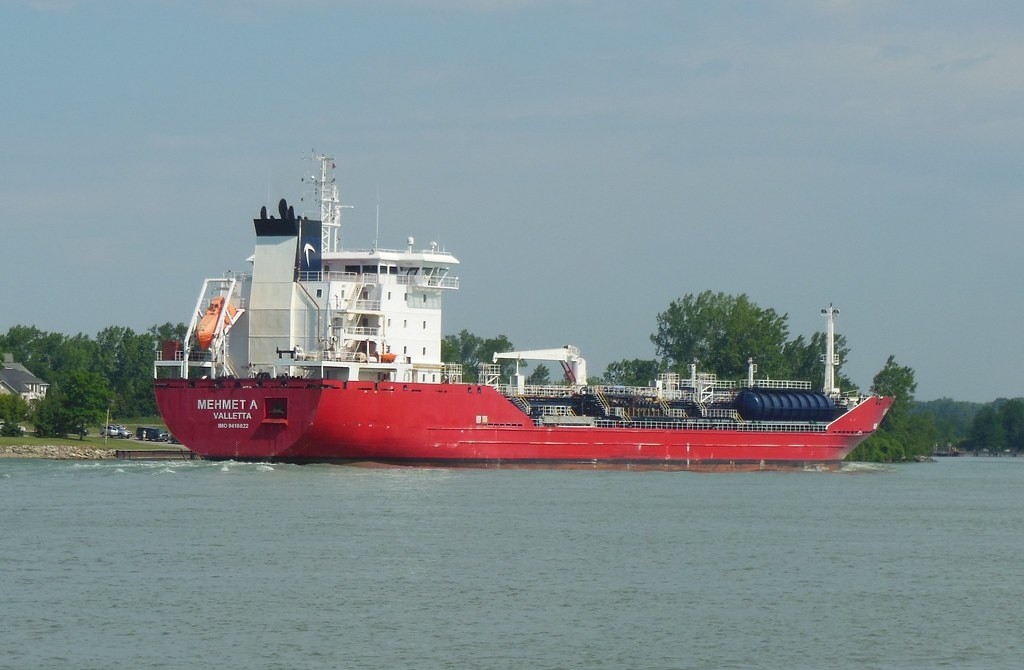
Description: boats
153,153,896,470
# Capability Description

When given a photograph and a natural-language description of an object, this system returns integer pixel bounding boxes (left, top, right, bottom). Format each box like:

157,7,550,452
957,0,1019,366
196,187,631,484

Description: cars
0,422,26,432
165,434,179,444
155,431,171,441
34,417,89,436
136,427,159,440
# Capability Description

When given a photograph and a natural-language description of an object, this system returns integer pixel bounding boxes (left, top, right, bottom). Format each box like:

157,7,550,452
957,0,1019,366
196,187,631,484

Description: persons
143,429,147,441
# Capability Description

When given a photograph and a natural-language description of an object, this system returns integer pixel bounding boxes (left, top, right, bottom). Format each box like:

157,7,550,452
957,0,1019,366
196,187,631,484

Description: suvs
101,425,132,439
